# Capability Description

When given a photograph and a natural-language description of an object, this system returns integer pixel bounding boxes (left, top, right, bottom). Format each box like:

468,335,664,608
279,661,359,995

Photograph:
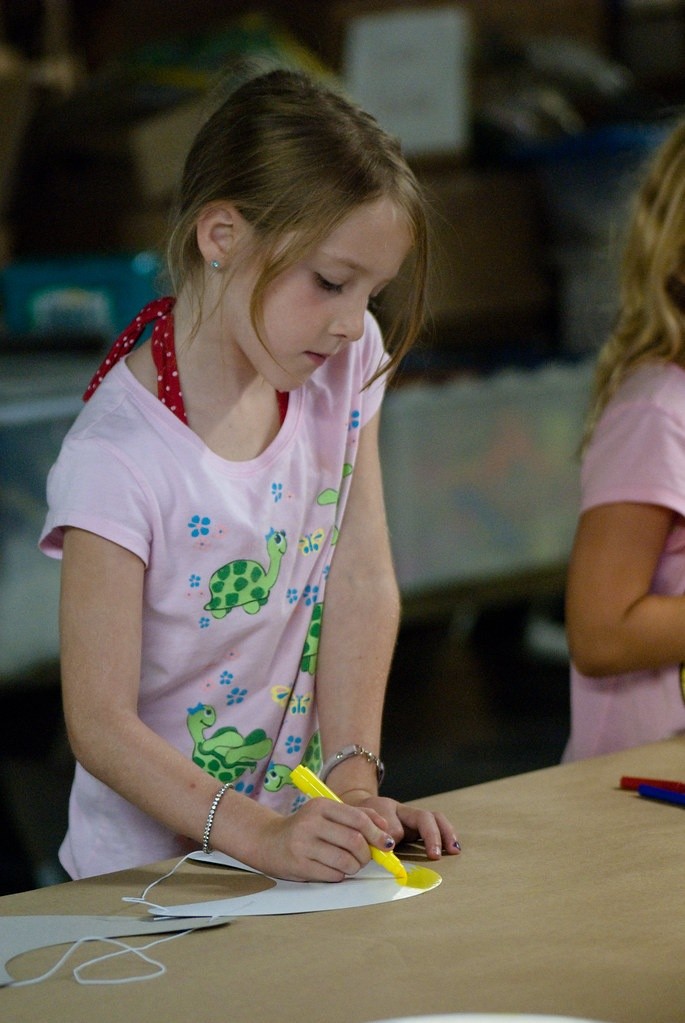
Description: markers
619,774,685,809
287,763,411,882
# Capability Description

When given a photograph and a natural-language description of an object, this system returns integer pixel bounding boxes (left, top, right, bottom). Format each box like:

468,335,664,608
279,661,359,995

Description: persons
563,123,685,763
464,37,599,243
242,13,339,98
2,56,142,262
42,70,460,884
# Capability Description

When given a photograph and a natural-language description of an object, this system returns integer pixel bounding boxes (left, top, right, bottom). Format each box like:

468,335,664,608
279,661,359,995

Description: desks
0,352,685,1023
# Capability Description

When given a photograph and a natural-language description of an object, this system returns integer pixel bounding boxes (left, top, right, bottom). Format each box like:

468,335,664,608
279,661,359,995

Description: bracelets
204,783,232,854
317,744,385,788
